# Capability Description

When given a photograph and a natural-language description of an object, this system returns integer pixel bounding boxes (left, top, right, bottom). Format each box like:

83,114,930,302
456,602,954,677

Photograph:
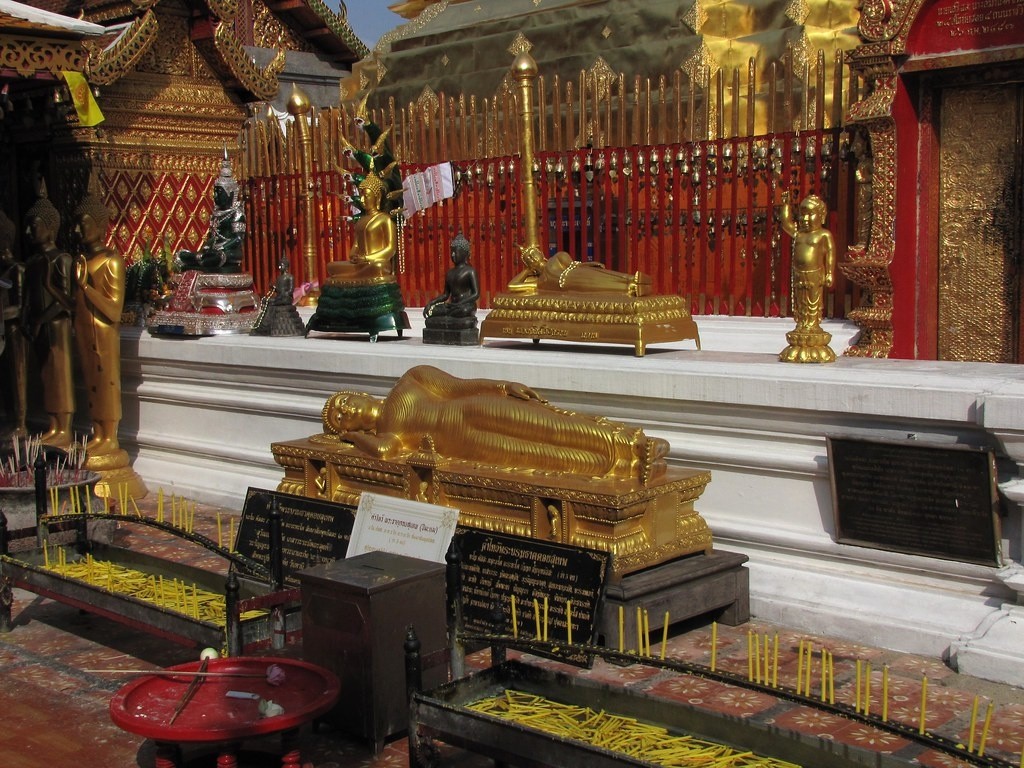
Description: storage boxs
299,549,449,758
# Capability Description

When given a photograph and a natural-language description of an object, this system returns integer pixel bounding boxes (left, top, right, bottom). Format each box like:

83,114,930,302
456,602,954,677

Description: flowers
169,647,218,724
81,662,288,685
253,700,285,724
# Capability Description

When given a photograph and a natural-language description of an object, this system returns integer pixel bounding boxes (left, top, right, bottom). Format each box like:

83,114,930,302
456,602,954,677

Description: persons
179,146,247,276
424,231,479,317
1,179,129,470
327,173,401,280
780,191,837,333
507,243,653,298
322,364,670,484
269,250,294,306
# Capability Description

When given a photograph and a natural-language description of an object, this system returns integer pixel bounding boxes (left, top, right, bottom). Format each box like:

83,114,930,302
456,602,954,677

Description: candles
463,594,994,768
34,477,269,630
